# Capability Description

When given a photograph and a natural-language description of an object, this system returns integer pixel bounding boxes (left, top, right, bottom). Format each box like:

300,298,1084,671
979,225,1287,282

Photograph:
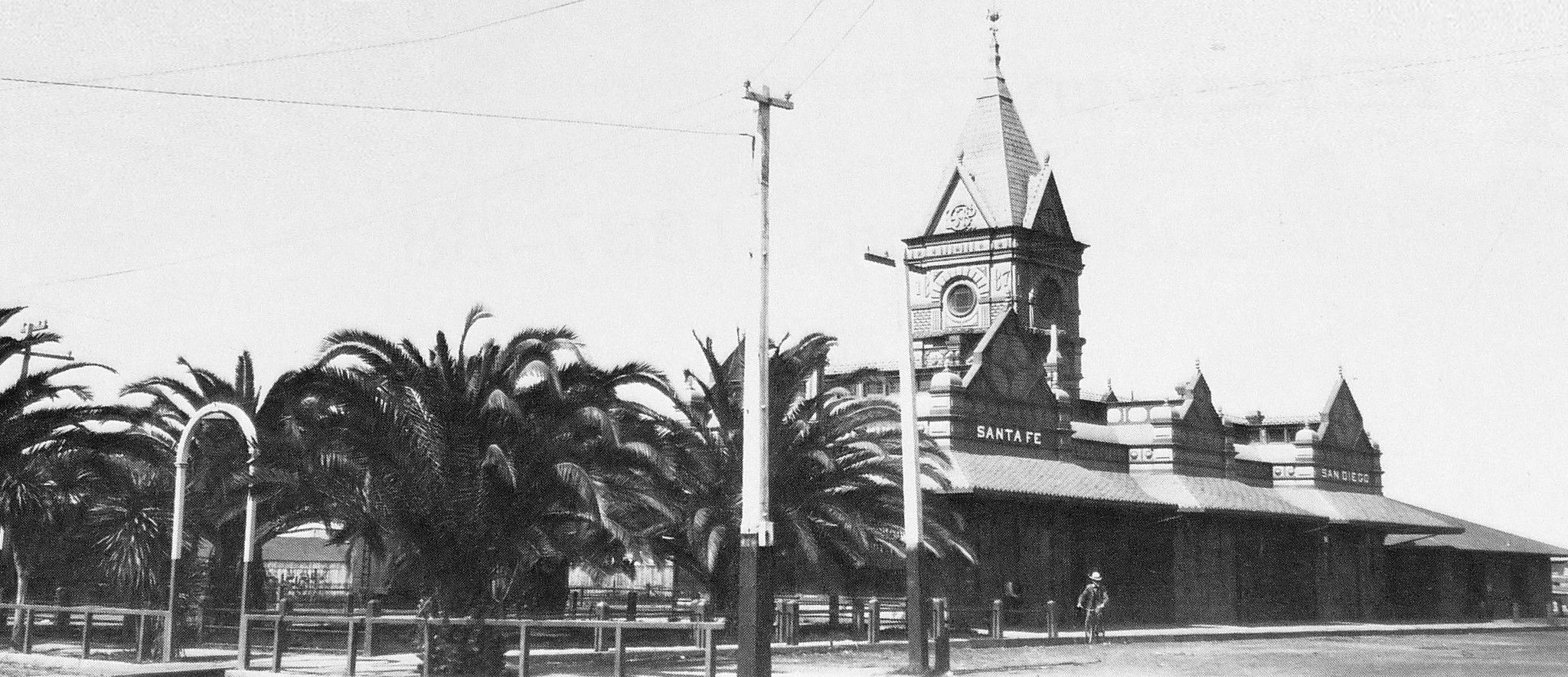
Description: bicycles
1081,607,1105,642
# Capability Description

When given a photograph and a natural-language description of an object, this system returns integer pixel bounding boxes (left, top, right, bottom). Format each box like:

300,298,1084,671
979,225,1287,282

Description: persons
1078,572,1109,633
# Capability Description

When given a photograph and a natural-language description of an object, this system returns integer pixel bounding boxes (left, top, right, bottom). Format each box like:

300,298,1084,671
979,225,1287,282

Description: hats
1088,572,1102,580
